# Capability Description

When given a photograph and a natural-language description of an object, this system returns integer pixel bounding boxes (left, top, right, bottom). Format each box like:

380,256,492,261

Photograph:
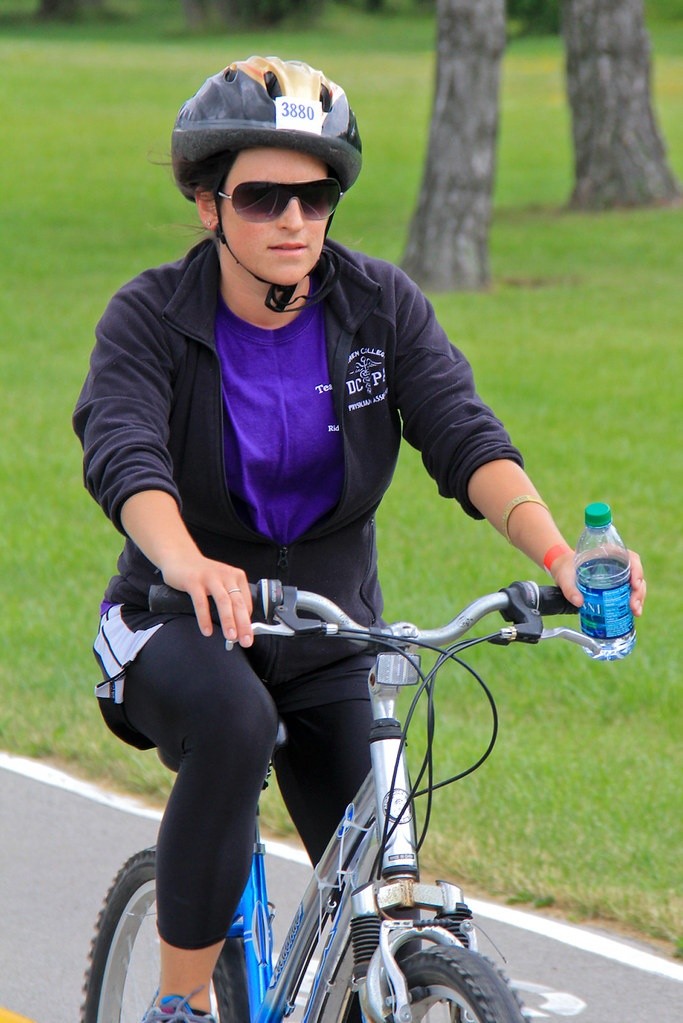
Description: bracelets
501,494,551,548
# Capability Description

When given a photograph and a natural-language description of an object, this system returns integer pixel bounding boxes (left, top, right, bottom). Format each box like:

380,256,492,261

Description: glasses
212,178,346,225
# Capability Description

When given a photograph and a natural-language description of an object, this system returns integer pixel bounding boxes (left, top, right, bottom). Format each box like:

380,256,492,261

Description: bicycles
79,580,582,1022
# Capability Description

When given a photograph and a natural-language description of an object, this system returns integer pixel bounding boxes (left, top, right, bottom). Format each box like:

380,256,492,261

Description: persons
68,53,653,1022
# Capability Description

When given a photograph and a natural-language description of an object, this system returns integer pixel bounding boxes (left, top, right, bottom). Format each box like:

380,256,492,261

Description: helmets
167,49,368,199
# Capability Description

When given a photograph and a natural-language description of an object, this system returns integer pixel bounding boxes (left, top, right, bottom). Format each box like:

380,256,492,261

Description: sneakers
140,991,222,1023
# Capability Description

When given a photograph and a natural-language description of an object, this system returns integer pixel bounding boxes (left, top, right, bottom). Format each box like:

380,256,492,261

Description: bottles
574,500,637,661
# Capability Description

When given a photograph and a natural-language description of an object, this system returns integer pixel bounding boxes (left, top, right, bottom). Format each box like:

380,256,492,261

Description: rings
227,588,241,594
